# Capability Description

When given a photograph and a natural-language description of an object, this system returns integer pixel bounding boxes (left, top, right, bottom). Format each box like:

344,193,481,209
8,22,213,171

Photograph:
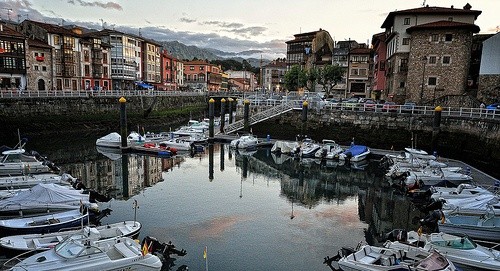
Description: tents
136,82,154,94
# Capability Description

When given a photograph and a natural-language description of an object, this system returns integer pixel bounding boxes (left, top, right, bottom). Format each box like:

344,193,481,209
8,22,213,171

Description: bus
207,72,229,93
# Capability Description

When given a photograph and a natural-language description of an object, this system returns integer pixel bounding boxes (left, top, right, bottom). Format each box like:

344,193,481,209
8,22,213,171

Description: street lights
421,56,428,106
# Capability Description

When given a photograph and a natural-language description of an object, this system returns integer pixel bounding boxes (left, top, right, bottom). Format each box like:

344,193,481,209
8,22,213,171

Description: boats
96,111,237,155
230,133,261,148
271,135,372,163
0,128,188,271
338,132,500,271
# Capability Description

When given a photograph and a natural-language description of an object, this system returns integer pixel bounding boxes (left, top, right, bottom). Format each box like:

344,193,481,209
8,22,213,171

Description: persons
87,85,98,97
479,100,496,118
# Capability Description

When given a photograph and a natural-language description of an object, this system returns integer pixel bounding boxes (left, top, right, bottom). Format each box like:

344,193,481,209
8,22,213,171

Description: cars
320,97,376,111
402,102,418,112
485,102,500,113
137,81,154,90
383,102,397,112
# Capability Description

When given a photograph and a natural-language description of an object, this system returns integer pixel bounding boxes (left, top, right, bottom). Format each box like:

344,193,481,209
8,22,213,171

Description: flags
142,240,148,257
203,246,207,259
